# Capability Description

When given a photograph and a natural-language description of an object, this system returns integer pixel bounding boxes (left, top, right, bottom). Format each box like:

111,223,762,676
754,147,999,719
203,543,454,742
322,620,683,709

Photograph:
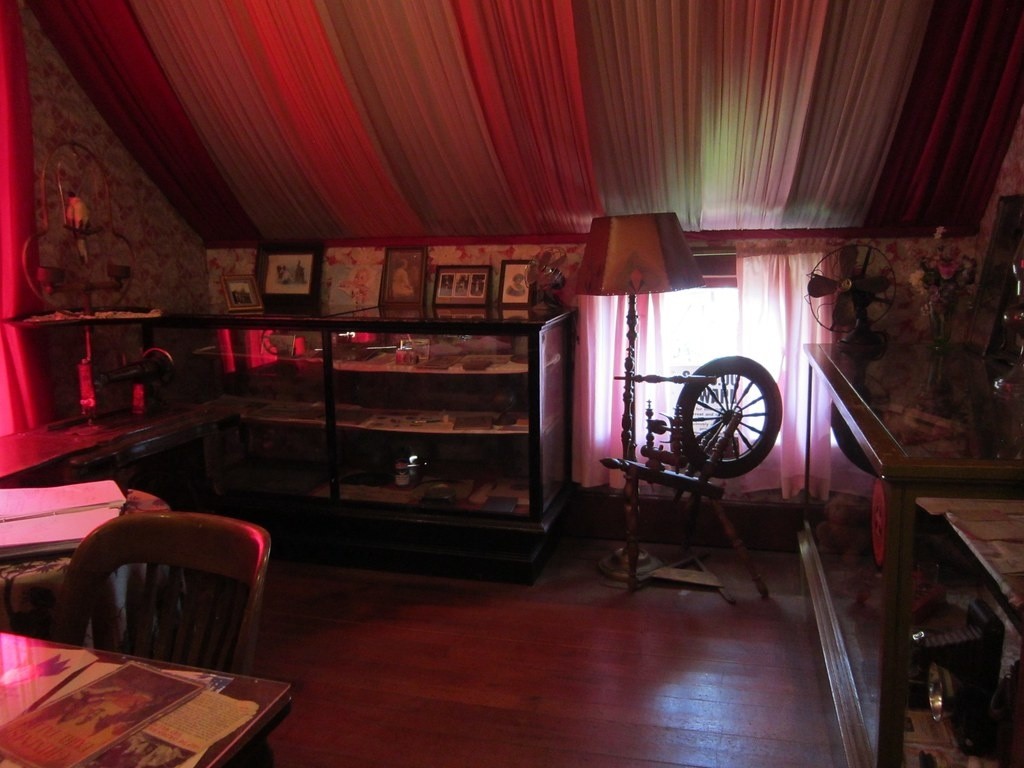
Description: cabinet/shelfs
134,301,581,587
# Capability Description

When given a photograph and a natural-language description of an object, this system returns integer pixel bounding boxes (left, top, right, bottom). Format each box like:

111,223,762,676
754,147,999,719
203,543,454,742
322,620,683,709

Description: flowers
906,224,984,315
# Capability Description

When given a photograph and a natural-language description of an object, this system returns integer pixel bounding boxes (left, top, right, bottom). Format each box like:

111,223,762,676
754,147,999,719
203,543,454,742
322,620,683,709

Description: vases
926,303,958,352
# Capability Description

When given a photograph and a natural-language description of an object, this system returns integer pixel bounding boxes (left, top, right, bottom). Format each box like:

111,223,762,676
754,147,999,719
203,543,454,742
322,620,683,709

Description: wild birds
65,192,92,265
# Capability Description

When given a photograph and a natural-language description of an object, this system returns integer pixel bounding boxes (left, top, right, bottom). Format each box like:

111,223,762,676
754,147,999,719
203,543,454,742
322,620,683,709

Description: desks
797,338,1024,764
0,396,249,525
0,629,297,768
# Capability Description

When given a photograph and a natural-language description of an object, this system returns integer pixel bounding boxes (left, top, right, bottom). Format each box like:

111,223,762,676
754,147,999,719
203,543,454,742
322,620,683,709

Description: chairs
44,510,272,676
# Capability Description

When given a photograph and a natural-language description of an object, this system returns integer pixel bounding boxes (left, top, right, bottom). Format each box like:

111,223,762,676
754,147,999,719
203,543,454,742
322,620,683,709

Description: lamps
570,212,709,581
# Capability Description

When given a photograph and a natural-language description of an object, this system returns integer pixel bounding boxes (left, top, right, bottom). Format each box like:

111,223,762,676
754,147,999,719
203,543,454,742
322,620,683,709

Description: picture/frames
497,259,537,309
380,246,427,309
255,241,324,311
432,264,492,306
220,274,264,312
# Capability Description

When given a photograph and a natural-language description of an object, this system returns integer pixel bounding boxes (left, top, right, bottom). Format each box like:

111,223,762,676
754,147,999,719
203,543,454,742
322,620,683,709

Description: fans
523,247,566,311
802,242,900,360
